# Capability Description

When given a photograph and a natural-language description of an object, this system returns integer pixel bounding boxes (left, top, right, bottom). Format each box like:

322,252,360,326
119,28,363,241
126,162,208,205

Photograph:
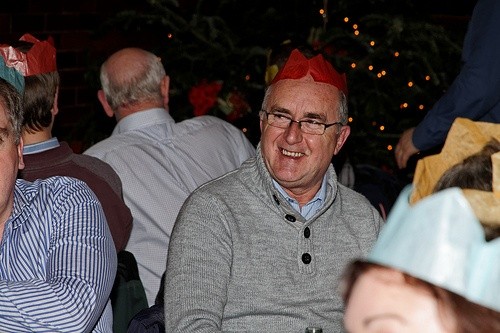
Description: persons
164,48,385,333
0,35,134,253
0,53,118,333
81,49,256,309
395,1,500,168
344,187,499,333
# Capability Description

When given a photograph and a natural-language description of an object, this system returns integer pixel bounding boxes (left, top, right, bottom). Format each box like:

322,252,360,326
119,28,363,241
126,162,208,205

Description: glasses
260,109,344,135
